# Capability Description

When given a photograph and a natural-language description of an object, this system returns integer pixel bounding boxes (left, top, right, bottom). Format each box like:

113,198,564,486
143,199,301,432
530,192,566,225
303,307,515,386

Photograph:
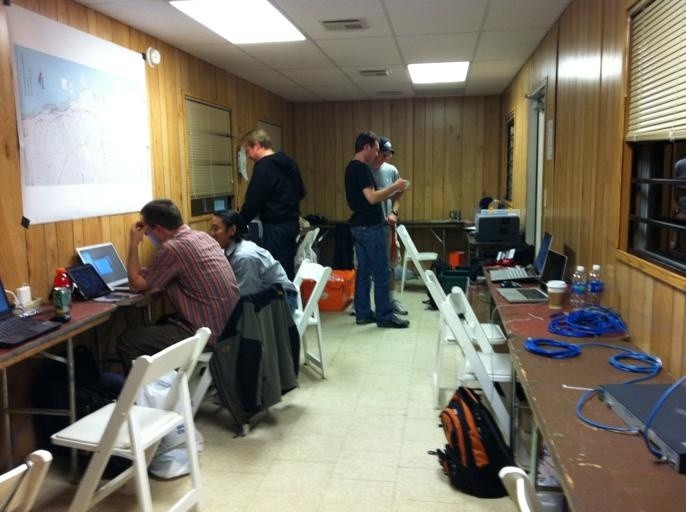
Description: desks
464,232,535,280
480,264,686,512
2,297,121,476
398,219,477,276
313,219,354,270
92,279,166,359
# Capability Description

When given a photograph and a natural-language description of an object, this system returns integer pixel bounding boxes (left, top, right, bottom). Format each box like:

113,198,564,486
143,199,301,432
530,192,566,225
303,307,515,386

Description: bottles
568,265,587,310
51,266,73,318
585,263,604,307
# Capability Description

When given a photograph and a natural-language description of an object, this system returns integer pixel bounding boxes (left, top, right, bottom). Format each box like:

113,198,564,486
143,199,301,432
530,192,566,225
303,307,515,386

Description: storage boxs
300,270,355,312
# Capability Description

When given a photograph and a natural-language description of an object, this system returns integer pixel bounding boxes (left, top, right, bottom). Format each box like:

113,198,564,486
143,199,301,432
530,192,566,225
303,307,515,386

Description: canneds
52,287,70,315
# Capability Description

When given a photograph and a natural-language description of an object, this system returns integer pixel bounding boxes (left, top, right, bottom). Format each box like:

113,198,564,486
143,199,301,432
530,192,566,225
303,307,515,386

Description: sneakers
350,300,357,315
391,301,408,314
378,315,409,329
356,311,377,325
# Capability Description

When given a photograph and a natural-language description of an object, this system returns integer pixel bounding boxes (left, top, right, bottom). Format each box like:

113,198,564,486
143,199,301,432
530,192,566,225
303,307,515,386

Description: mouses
49,312,69,323
501,280,521,288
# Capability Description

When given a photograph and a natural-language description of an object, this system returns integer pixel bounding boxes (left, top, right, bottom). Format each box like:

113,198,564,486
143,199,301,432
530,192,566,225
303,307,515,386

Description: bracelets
391,209,398,215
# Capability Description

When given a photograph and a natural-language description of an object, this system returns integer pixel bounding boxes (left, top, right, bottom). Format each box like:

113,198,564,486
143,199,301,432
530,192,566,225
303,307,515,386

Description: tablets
67,264,109,300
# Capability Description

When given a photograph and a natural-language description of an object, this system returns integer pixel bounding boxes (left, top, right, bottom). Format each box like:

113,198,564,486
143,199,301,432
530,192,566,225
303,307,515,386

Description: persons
209,209,299,316
236,129,306,282
349,136,410,316
118,199,244,383
345,131,409,328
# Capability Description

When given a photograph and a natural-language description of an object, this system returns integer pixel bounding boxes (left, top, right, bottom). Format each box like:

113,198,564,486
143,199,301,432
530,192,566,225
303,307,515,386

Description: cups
448,210,455,221
455,210,462,222
546,279,567,309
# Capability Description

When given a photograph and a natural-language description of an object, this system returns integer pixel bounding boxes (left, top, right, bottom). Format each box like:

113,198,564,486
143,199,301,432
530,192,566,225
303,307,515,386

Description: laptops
80,243,131,291
0,280,60,347
489,232,553,281
496,250,568,303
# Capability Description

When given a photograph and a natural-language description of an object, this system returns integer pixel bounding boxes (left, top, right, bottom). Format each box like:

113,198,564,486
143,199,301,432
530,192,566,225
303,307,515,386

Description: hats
378,135,396,155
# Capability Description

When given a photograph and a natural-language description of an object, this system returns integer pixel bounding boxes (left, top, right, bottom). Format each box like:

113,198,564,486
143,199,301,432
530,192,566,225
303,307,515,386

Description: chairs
0,443,54,512
293,226,321,268
440,285,523,447
288,257,333,383
48,325,212,512
391,223,439,297
422,268,507,405
190,281,287,440
498,460,571,512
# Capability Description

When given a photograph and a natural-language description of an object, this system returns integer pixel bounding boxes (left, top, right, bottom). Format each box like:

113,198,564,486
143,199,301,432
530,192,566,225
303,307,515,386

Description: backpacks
427,384,520,499
